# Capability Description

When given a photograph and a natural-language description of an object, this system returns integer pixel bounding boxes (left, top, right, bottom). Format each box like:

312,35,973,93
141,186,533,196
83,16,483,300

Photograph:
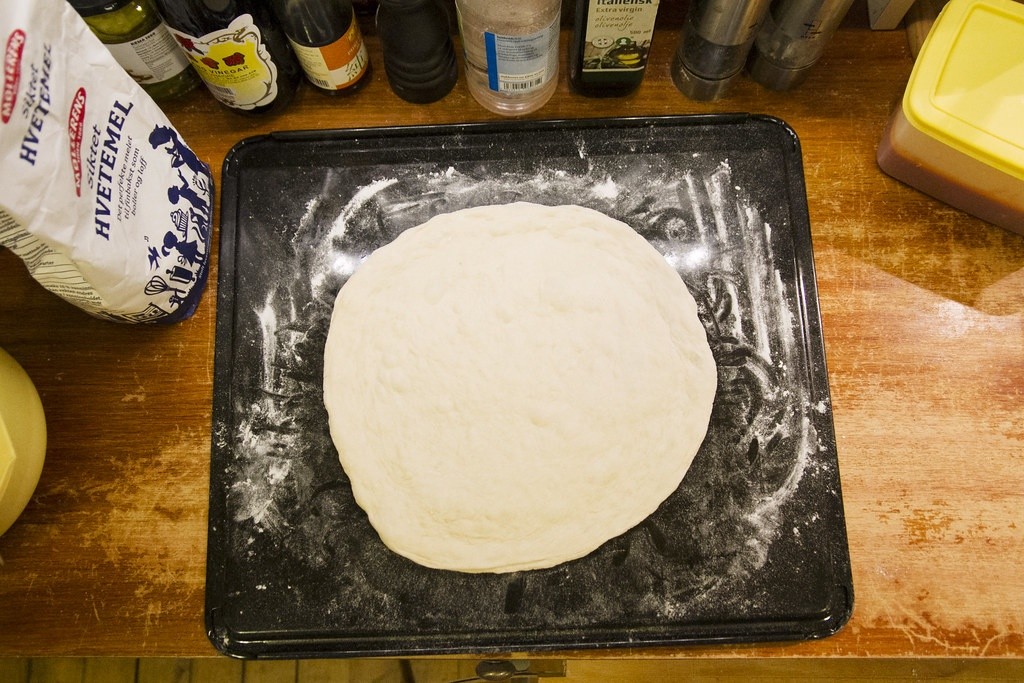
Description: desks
0,22,1024,682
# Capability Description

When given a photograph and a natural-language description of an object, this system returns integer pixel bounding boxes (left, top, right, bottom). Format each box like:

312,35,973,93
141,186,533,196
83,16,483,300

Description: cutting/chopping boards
203,113,856,659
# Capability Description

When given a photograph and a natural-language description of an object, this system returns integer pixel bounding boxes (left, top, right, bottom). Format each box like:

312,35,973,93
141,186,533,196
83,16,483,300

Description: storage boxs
876,0,1024,237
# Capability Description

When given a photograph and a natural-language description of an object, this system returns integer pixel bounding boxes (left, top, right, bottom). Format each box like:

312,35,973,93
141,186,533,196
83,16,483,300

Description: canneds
67,0,203,100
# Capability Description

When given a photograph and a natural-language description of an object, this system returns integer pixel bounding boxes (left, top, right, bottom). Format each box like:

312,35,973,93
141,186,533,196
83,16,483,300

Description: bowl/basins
874,0,1024,234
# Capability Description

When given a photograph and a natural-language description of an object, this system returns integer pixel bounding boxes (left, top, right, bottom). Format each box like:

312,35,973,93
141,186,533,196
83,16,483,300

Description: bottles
148,0,305,124
673,0,770,99
568,0,662,98
66,0,202,101
282,0,373,98
453,0,560,116
748,0,854,92
375,0,458,103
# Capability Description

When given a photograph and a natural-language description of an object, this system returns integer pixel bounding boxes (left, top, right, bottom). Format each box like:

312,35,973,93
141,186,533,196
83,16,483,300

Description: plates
1,349,47,534
